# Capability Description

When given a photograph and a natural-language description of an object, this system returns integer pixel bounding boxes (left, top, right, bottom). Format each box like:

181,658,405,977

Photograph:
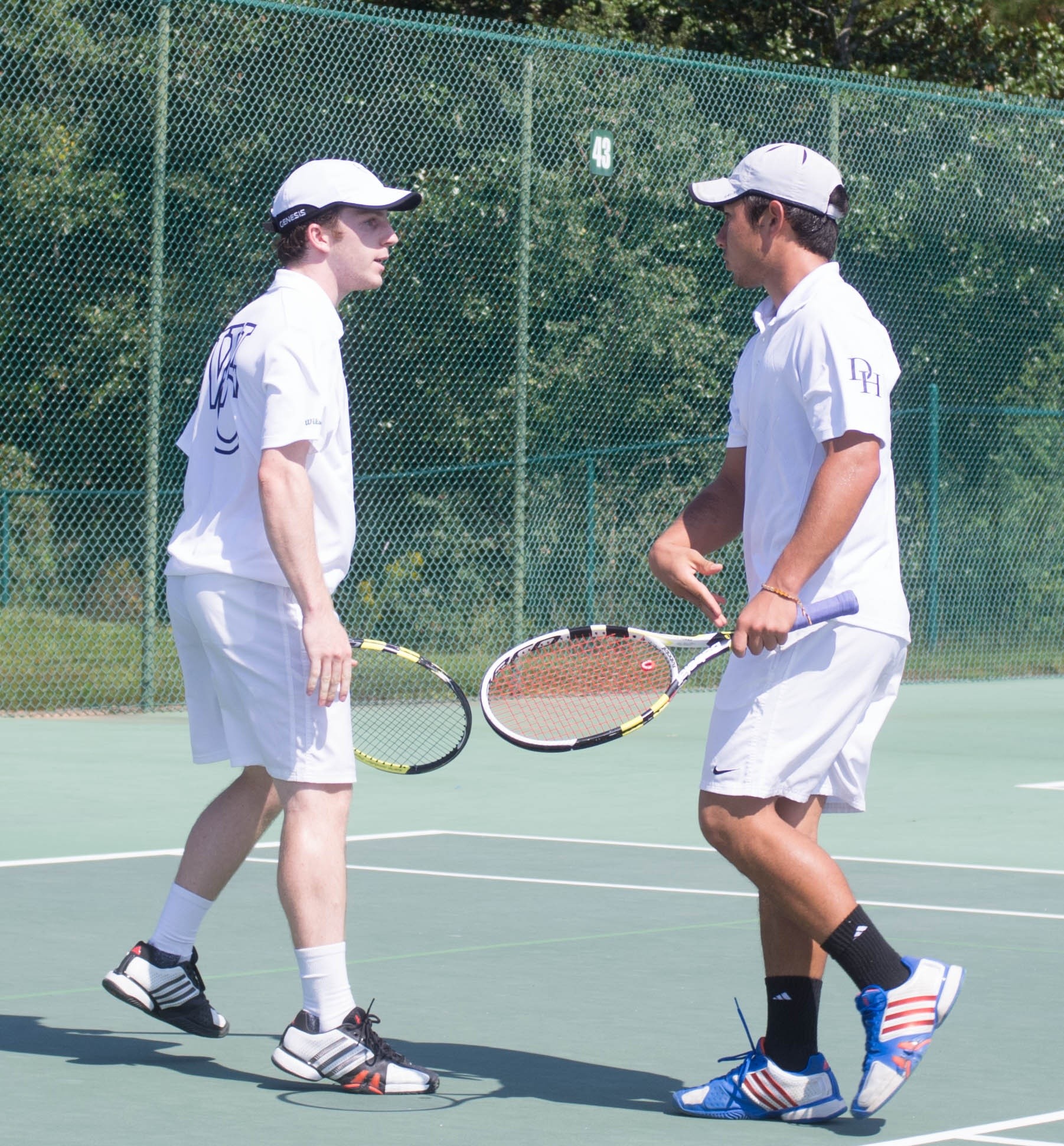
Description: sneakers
102,940,230,1038
271,998,440,1094
850,955,966,1119
667,997,848,1124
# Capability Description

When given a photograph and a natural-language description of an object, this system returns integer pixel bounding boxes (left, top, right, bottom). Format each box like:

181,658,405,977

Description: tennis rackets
349,633,473,776
479,589,862,754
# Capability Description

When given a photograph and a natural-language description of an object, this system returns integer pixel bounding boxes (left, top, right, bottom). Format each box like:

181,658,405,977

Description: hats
270,159,422,233
688,143,850,224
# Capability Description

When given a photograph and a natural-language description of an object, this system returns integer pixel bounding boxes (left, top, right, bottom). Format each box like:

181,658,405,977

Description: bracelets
762,582,812,625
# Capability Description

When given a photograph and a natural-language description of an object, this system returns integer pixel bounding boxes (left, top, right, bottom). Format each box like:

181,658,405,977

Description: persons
103,161,441,1096
646,144,966,1128
